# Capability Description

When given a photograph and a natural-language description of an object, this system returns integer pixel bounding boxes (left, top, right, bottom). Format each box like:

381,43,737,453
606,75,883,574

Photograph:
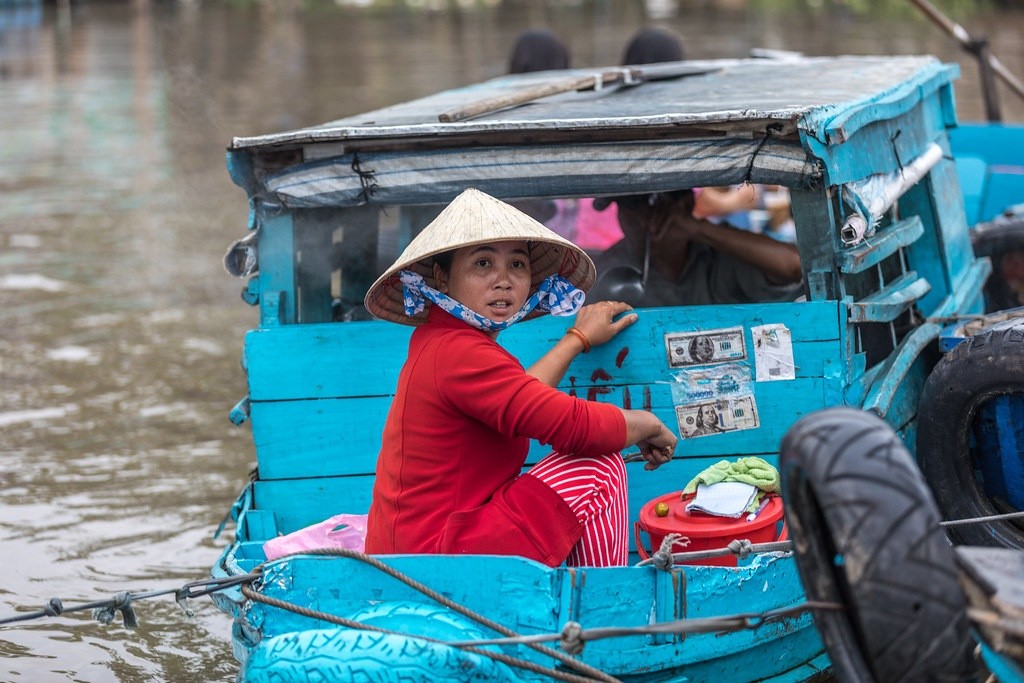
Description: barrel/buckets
634,489,787,567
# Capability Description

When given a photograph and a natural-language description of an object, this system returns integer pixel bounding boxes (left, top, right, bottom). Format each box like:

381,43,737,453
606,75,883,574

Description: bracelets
567,328,591,353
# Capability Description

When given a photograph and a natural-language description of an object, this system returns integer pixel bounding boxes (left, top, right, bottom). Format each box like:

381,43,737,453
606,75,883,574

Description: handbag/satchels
261,513,368,560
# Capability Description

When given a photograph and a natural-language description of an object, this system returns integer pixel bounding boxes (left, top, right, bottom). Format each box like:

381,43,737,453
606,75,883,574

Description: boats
205,48,1023,683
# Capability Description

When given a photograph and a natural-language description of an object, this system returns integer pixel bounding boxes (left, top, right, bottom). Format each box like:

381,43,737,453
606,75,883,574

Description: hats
363,188,597,327
414,198,557,236
592,188,696,214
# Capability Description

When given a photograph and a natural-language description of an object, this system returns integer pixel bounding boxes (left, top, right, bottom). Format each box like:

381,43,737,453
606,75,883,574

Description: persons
494,25,812,306
363,186,679,566
